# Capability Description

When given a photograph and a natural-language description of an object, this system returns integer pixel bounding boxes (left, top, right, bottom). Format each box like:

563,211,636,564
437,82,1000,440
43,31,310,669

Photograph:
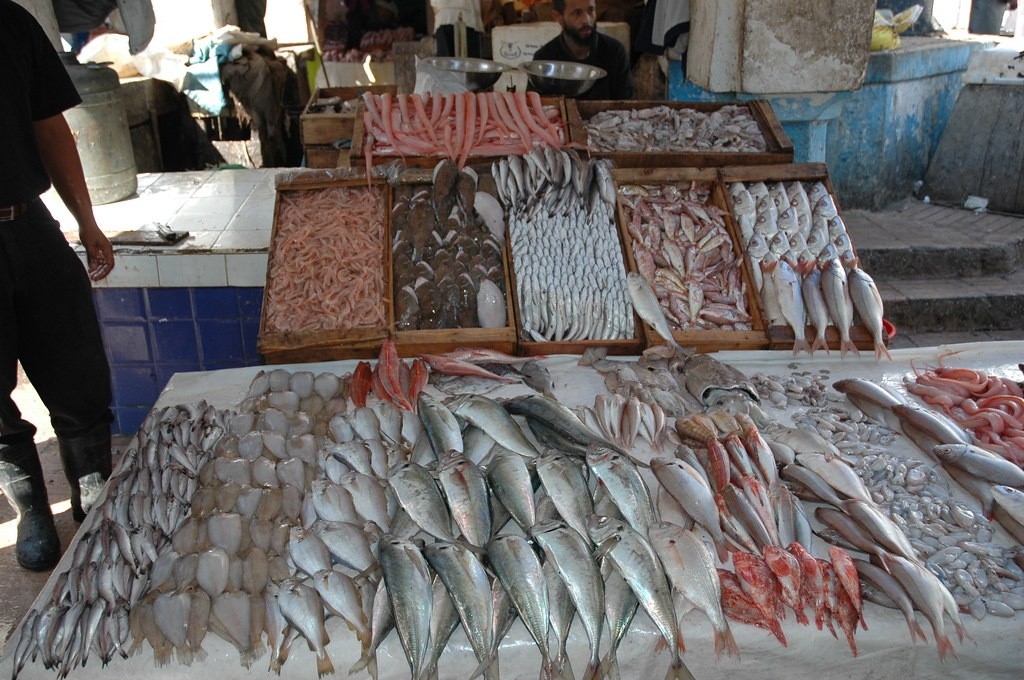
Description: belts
0,200,37,221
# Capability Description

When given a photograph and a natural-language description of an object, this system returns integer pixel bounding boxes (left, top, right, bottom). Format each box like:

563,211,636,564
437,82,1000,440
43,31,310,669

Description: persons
525,0,636,99
431,1,486,58
0,1,117,572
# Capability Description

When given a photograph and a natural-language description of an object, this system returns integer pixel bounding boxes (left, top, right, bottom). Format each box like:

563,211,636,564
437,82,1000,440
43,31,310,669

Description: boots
0,436,62,571
54,423,112,522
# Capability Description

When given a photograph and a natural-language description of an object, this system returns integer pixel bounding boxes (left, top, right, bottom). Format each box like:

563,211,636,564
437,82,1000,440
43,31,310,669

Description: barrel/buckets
56,52,138,206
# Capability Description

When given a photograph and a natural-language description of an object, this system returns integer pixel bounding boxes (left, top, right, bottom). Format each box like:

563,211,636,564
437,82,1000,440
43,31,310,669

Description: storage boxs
492,21,630,69
316,61,395,88
256,85,889,366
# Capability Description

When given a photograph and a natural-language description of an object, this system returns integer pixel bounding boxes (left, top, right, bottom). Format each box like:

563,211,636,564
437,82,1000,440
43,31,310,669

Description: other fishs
12,340,1024,680
264,146,893,363
581,106,767,154
